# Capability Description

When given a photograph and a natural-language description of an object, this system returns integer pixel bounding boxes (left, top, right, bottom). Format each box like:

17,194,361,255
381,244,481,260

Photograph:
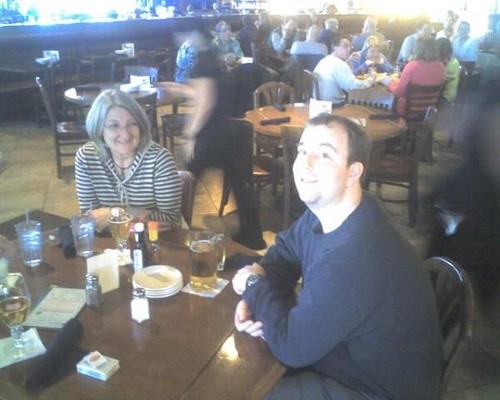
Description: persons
176,0,500,122
232,112,445,400
171,16,264,250
76,88,190,230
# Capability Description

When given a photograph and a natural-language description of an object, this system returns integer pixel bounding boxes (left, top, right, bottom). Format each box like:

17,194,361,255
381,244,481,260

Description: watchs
246,272,265,289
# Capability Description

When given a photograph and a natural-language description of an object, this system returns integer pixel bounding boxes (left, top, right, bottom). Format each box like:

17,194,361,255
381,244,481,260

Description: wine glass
107,212,133,263
0,273,35,358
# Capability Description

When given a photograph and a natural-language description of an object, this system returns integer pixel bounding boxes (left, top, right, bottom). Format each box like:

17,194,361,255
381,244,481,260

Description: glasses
103,121,140,131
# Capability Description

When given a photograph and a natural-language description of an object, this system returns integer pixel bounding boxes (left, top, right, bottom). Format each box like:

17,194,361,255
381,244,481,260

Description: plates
132,264,184,299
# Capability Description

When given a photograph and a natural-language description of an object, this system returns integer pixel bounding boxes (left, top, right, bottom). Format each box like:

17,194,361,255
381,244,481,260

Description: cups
15,219,43,267
189,233,226,292
70,215,95,256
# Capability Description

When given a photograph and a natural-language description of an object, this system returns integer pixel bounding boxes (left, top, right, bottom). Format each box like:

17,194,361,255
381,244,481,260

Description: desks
244,103,408,142
346,74,399,111
63,82,157,105
0,226,297,400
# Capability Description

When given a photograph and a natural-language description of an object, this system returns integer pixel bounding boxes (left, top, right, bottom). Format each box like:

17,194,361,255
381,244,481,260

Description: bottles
147,230,161,265
84,272,103,307
130,222,147,274
130,287,150,324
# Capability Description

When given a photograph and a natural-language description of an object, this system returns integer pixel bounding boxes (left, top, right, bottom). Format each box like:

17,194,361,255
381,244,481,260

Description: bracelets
184,134,197,139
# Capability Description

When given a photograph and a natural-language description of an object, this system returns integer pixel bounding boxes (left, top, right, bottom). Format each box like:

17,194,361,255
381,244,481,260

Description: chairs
178,170,197,228
425,256,476,400
35,50,481,227
